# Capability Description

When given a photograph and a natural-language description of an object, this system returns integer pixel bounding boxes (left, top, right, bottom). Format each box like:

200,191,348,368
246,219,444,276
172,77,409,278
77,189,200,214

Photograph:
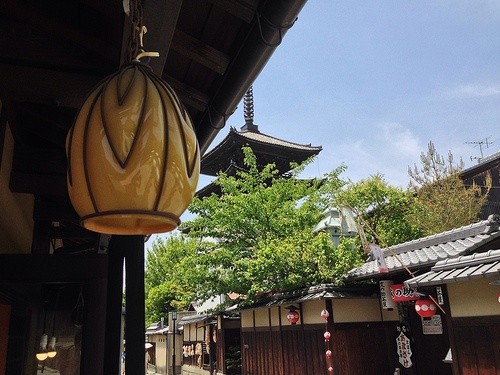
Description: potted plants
224,344,242,375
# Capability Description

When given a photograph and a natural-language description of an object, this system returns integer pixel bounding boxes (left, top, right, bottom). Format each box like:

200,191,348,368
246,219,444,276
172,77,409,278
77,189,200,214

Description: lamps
65,1,202,235
285,305,300,325
47,309,57,358
411,289,438,321
35,307,48,361
